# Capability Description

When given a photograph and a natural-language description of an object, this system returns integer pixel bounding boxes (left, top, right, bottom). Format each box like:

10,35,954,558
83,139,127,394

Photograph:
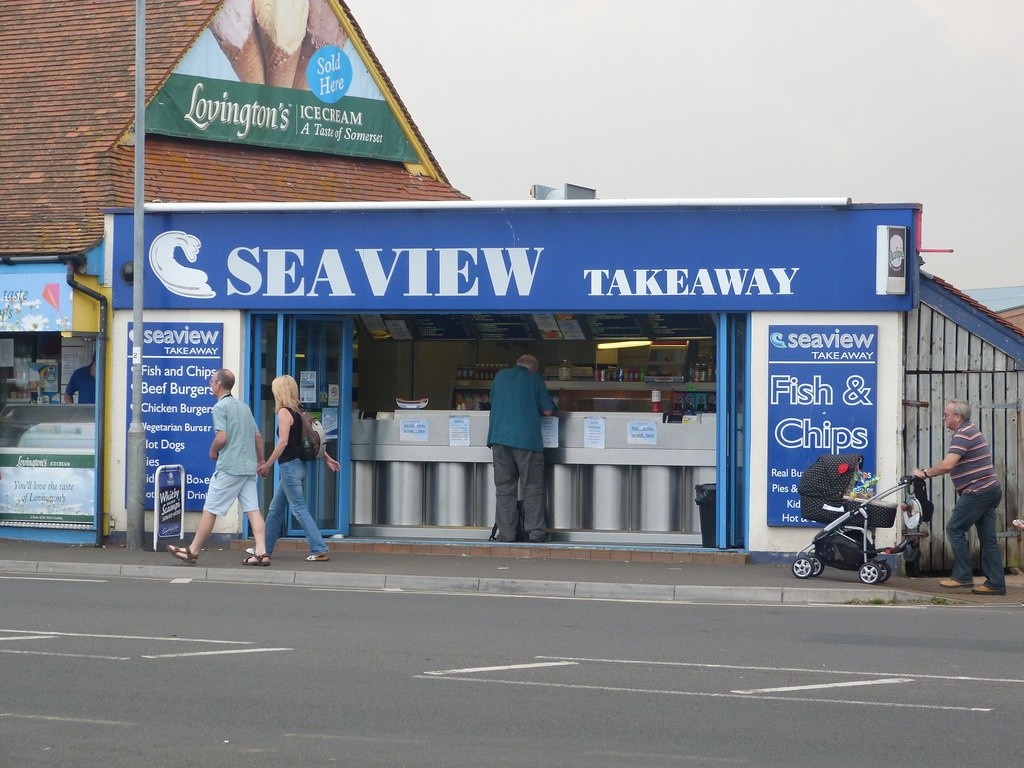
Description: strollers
789,453,922,586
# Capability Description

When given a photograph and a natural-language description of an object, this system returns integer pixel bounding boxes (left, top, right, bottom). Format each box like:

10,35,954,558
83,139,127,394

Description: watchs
922,468,930,478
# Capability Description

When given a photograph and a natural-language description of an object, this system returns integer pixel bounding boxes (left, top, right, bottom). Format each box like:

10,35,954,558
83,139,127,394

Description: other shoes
496,534,516,542
529,534,551,543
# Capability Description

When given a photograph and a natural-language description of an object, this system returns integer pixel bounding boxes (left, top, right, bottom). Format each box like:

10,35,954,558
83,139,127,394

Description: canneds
594,369,605,381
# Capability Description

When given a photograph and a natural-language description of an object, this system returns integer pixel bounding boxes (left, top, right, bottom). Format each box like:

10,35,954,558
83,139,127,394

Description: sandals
166,543,198,566
241,550,271,566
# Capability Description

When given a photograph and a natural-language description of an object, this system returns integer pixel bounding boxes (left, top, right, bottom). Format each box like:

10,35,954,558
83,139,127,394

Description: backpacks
284,404,326,460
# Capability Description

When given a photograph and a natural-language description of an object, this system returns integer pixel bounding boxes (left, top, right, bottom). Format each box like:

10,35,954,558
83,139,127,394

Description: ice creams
207,0,349,92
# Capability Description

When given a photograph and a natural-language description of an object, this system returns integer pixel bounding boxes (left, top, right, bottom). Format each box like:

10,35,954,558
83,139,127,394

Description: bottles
456,361,509,381
693,351,714,383
558,359,572,381
13,356,31,380
15,384,28,401
619,365,646,383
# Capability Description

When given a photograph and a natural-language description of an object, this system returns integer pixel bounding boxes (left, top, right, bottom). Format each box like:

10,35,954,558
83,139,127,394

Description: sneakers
972,585,1005,595
939,579,973,588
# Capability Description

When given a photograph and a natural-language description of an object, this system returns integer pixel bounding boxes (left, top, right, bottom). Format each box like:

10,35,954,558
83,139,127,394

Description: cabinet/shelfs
0,404,96,544
644,341,699,383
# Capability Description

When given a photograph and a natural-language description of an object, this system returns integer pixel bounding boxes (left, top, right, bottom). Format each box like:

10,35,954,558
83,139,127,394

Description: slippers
245,547,271,559
305,554,329,561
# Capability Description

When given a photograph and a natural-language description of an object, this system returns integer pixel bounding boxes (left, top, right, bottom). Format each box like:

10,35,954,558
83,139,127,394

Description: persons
486,354,554,543
914,401,1006,595
165,370,271,567
64,352,96,404
246,375,340,561
1013,520,1024,607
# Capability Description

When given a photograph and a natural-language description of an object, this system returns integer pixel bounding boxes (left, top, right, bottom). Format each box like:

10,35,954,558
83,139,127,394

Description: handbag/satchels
913,477,933,522
489,499,548,542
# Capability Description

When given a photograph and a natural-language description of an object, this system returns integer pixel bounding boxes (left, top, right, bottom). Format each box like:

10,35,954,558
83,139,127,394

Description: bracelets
260,463,266,465
326,458,330,463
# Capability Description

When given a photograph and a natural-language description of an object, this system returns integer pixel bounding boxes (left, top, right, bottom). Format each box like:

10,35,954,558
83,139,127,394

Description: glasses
944,413,959,418
209,379,223,386
